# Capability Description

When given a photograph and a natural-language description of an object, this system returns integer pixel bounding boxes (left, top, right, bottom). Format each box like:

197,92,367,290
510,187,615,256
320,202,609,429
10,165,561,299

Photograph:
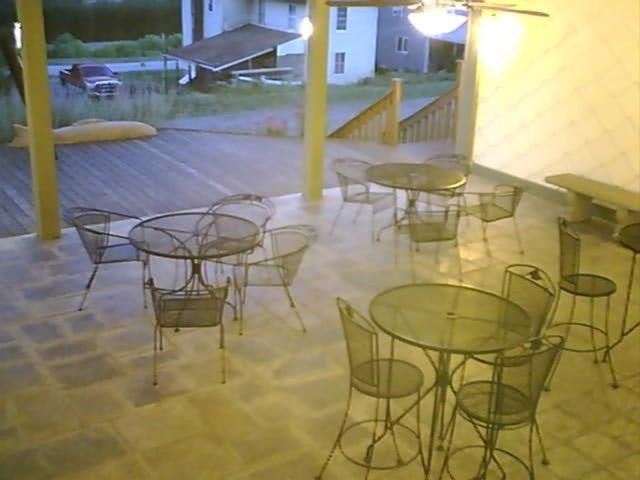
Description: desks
364,162,467,252
128,212,258,332
603,222,639,361
369,284,550,480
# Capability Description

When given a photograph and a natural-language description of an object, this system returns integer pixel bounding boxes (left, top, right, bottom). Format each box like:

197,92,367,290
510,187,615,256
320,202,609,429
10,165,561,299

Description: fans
324,0,549,18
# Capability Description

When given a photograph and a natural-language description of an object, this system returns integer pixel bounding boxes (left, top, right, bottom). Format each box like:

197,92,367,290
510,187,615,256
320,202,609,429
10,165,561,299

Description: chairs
233,224,317,336
442,264,556,466
414,152,473,221
59,205,157,309
439,335,564,480
312,296,428,480
328,157,397,243
394,203,466,282
143,278,230,386
531,215,618,389
195,192,277,304
456,183,526,257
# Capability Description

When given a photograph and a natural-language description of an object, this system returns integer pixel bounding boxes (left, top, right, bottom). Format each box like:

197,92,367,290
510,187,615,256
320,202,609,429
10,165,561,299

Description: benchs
545,172,640,240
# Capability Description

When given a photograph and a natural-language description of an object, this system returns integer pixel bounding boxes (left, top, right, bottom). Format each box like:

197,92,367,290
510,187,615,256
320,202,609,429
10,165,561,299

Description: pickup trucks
59,62,123,98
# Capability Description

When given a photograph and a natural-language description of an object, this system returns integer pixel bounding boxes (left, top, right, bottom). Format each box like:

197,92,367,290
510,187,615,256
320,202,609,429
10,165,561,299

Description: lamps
404,5,467,37
